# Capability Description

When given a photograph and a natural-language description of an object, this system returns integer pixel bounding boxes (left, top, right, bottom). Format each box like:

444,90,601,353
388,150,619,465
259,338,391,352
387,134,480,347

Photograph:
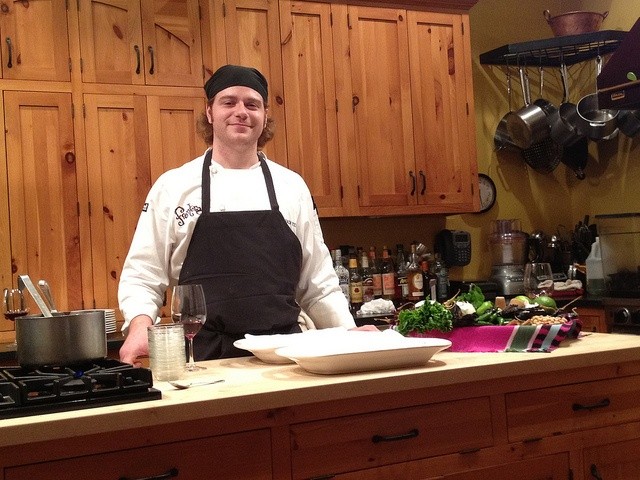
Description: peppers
476,310,504,326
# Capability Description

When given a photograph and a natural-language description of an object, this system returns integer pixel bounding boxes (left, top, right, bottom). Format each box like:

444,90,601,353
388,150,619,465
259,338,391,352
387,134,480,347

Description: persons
118,66,382,370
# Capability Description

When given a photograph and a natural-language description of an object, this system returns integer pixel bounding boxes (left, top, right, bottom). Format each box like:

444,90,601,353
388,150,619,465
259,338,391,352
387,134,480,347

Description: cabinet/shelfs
225,1,342,211
0,410,276,479
72,0,207,89
283,361,638,480
93,92,213,323
0,88,82,336
346,1,478,209
1,1,71,85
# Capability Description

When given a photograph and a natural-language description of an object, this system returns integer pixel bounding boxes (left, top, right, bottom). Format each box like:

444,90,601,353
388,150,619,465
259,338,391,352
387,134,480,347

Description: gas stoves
600,293,640,333
0,358,162,421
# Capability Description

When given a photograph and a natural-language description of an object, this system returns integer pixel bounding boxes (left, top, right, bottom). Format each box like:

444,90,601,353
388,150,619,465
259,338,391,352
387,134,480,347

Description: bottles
363,247,382,304
407,243,426,304
349,246,364,310
395,245,410,301
422,259,438,303
585,236,604,296
330,246,349,315
381,245,395,301
434,252,449,301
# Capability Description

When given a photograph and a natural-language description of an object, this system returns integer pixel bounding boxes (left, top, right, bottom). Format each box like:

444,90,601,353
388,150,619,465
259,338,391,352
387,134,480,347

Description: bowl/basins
148,322,186,384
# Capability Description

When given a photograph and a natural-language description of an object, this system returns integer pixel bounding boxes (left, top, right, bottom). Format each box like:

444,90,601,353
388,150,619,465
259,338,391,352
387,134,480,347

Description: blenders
488,220,529,297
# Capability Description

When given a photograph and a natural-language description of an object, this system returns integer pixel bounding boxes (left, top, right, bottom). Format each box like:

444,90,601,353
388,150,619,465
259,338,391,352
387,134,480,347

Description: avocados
507,295,559,311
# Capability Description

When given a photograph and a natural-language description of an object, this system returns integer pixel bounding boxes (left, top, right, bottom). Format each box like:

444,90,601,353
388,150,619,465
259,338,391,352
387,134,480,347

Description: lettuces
455,286,485,309
398,294,453,336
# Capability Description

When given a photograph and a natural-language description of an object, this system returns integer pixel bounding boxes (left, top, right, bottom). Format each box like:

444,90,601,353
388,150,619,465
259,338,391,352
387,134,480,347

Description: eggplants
503,304,555,320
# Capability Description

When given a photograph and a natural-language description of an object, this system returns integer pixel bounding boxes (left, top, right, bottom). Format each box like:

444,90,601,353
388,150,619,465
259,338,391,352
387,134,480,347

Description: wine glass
523,262,554,302
1,289,31,351
169,283,207,374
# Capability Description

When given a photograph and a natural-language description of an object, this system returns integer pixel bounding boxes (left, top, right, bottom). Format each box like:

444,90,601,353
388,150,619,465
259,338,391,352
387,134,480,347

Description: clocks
478,172,497,215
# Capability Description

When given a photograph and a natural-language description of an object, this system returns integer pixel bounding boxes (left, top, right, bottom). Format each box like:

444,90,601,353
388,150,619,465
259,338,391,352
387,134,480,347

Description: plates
273,335,452,374
233,328,352,365
100,306,118,335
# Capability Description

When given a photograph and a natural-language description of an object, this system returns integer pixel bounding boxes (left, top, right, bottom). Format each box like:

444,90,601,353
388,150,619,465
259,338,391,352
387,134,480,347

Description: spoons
37,279,61,318
530,227,546,245
550,232,559,243
625,72,638,83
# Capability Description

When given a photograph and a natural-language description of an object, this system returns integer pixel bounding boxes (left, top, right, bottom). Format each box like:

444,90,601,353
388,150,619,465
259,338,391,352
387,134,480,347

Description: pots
556,135,589,181
14,310,109,372
489,62,620,152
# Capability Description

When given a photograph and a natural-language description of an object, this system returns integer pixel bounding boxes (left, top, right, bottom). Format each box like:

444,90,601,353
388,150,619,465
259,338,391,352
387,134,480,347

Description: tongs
17,275,59,318
